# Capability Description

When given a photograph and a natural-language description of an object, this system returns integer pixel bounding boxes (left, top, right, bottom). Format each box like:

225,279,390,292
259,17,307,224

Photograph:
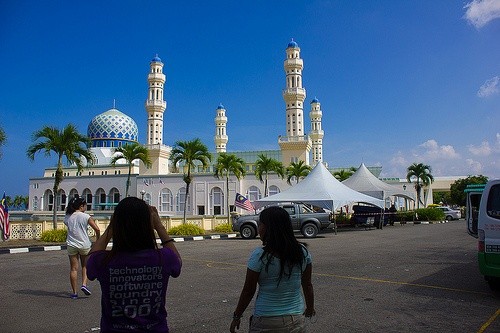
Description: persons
62,197,100,299
83,197,182,333
229,206,316,333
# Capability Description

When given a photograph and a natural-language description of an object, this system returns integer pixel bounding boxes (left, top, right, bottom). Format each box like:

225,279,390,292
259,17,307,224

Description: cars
427,204,441,209
436,207,462,221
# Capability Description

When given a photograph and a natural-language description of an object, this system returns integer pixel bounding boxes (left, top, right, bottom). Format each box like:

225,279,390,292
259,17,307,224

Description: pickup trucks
233,202,332,239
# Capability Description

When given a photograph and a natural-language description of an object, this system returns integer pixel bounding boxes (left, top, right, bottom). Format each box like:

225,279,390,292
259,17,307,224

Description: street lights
403,184,407,211
140,190,146,200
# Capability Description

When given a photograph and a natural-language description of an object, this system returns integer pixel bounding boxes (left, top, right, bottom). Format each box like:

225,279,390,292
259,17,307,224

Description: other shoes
81,286,92,296
70,293,79,299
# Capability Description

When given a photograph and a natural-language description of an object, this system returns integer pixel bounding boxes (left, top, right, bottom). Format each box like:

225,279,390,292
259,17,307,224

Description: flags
0,192,11,240
234,193,254,213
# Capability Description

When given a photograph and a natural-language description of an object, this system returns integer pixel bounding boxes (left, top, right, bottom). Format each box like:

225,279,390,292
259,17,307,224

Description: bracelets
232,312,243,320
160,238,174,245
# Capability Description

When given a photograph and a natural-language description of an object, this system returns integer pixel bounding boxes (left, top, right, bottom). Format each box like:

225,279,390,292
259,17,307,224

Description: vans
465,177,500,288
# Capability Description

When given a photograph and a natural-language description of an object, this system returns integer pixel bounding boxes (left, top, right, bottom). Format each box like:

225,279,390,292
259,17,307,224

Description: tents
254,161,385,235
340,163,416,228
